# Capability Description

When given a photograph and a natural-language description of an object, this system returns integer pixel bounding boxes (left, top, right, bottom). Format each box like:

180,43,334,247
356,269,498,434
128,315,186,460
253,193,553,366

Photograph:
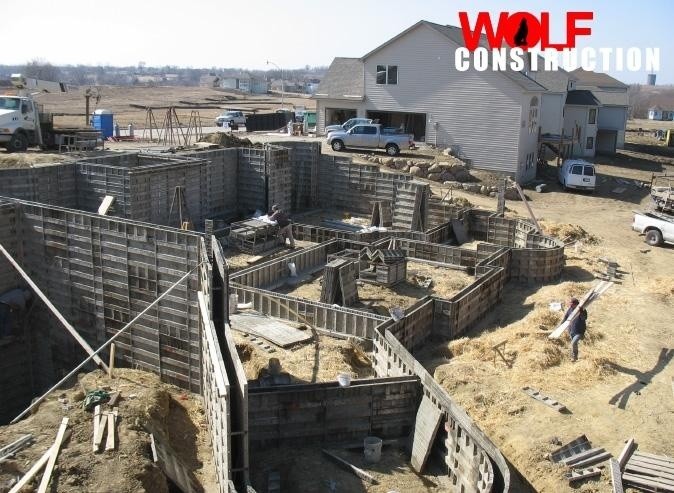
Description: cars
295,111,307,123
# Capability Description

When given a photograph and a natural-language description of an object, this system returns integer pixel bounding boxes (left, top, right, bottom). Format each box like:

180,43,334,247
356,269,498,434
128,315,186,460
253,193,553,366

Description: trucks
0,95,102,151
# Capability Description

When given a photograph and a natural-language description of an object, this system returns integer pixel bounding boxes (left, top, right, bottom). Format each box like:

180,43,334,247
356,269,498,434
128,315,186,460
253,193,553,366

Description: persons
557,297,587,362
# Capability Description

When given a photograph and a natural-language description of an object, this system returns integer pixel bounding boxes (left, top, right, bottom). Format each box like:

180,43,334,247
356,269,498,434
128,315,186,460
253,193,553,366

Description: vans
558,159,596,192
274,108,292,113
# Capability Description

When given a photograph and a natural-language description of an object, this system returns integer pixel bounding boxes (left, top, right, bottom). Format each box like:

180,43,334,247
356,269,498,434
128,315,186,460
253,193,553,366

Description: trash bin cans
656,130,663,139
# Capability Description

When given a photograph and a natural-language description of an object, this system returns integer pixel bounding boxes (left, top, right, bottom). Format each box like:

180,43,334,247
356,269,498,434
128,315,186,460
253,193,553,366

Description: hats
568,298,578,306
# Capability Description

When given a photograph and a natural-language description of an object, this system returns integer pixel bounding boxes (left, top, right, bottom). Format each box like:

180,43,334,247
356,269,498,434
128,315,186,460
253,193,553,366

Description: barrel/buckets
575,242,582,252
338,373,351,387
364,436,382,464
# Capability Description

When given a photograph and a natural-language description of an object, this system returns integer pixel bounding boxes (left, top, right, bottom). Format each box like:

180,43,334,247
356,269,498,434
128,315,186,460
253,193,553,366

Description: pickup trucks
631,209,674,246
324,118,415,156
215,110,247,126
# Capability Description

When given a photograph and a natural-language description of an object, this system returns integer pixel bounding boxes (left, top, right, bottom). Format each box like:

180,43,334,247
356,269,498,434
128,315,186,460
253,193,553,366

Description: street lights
266,59,283,108
